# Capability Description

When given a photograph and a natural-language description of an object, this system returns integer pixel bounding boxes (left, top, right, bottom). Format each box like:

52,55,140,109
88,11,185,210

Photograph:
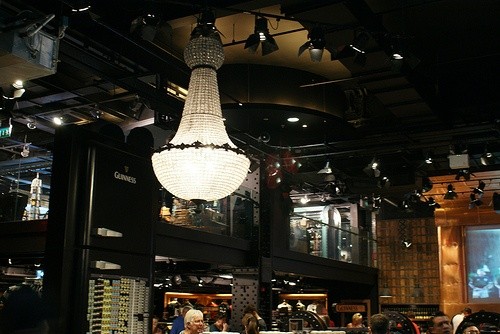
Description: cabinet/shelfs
381,303,439,334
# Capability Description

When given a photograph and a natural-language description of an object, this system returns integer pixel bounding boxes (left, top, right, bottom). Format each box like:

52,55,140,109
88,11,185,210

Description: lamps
91,104,103,118
129,96,144,117
27,115,36,129
20,144,30,157
150,0,500,215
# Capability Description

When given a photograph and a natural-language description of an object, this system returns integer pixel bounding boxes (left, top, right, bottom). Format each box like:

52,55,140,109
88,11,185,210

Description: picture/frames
461,225,500,305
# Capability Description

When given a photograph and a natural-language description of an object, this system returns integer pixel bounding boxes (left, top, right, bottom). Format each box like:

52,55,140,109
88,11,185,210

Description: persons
151,297,267,334
346,307,481,334
